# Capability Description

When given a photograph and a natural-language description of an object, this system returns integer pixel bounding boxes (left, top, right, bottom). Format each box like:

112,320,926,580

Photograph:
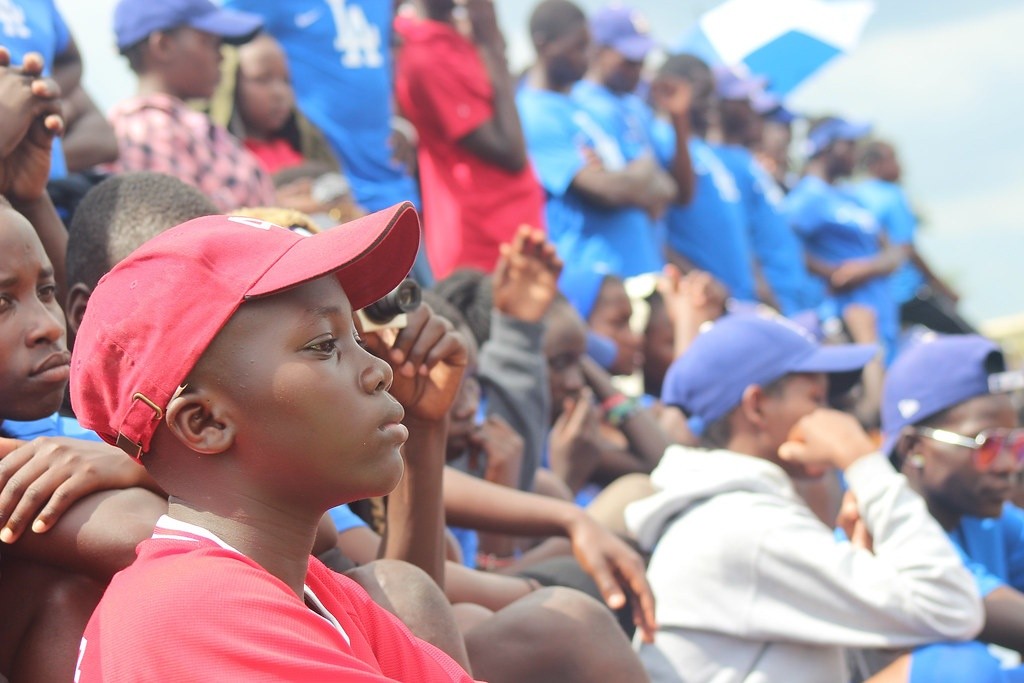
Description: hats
588,5,658,62
660,305,878,438
880,336,1024,460
806,116,872,157
714,63,809,125
112,0,265,56
69,199,421,465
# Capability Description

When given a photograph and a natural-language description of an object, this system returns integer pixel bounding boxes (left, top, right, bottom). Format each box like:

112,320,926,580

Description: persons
0,1,1023,683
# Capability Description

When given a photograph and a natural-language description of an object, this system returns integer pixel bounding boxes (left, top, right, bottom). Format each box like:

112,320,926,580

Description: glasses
903,425,1024,470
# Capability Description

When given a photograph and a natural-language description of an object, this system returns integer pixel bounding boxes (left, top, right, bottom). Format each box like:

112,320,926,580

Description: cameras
352,278,422,333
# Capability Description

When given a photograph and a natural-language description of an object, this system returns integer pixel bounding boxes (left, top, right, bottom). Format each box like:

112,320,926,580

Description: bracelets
607,397,637,424
602,393,626,411
